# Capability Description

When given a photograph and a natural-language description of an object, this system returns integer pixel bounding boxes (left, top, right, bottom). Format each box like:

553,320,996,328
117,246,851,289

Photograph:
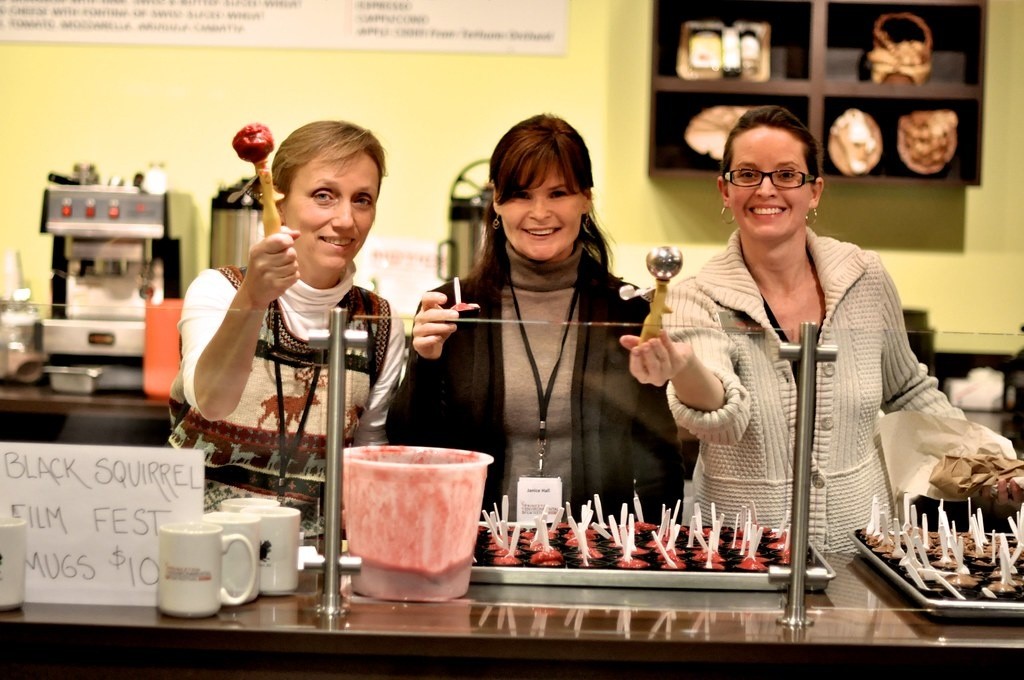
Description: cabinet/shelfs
648,0,986,184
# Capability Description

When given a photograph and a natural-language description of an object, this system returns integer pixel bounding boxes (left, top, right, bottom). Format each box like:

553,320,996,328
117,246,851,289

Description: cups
0,518,28,610
252,507,302,596
157,522,256,618
204,510,262,603
221,497,281,514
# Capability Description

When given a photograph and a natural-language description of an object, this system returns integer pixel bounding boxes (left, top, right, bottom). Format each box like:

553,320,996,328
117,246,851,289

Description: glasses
725,171,817,189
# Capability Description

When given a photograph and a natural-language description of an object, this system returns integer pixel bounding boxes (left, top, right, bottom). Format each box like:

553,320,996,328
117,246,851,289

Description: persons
387,115,685,528
170,121,406,542
619,105,1024,552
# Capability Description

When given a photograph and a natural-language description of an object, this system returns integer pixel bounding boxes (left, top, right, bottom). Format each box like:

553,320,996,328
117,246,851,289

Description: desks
0,378,174,420
0,550,1023,679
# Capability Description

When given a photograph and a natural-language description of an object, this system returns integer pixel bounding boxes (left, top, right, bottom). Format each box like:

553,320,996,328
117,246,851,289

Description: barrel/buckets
342,445,496,602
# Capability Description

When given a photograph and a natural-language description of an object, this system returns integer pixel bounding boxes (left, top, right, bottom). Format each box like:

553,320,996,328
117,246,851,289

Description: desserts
449,302,480,331
857,523,1024,605
474,520,796,571
